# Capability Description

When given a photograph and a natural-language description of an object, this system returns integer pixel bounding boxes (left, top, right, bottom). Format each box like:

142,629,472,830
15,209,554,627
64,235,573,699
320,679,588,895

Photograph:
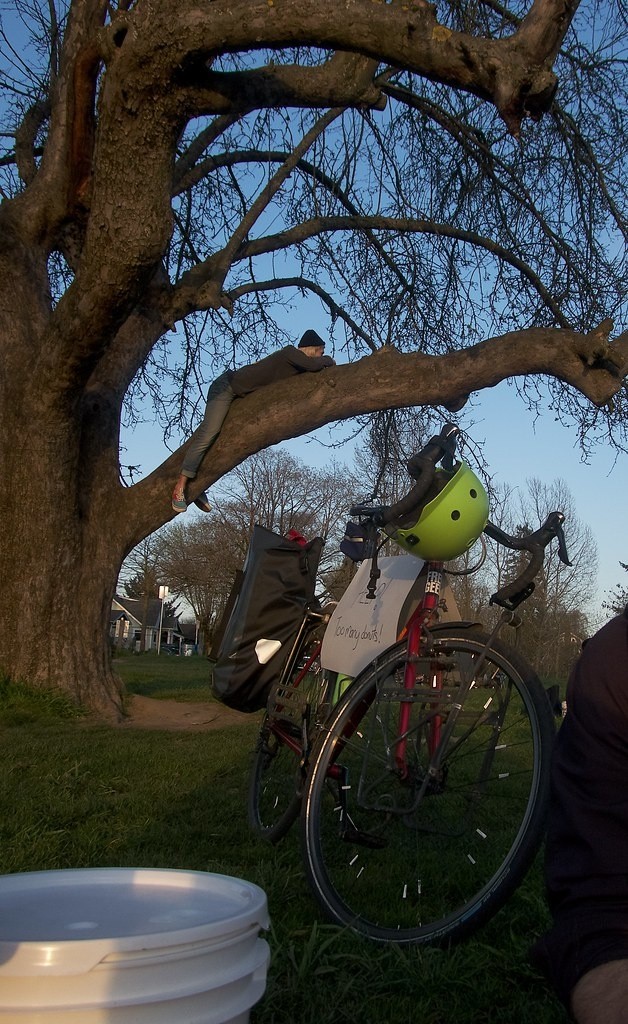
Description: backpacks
209,522,327,713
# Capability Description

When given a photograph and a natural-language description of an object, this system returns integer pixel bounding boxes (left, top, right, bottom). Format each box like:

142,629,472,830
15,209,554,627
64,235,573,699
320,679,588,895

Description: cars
154,642,179,656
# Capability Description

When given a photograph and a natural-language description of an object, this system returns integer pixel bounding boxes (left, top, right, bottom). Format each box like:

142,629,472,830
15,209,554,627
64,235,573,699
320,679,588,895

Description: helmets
385,463,488,563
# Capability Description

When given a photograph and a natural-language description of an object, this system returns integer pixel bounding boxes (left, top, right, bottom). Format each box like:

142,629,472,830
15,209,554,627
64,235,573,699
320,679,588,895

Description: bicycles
247,430,572,951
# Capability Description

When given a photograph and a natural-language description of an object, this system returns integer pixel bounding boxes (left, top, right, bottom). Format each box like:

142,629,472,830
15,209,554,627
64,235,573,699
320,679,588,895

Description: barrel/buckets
0,865,274,1024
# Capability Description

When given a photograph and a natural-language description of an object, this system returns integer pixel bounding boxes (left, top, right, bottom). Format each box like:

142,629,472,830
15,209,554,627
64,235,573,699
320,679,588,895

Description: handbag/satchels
322,535,462,679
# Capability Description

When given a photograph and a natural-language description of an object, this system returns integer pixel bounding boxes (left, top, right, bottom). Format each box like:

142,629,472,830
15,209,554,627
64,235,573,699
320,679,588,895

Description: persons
543,605,628,1024
173,329,337,513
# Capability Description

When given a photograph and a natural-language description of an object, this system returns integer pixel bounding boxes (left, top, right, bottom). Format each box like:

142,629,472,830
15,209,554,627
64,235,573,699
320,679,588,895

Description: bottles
332,672,353,705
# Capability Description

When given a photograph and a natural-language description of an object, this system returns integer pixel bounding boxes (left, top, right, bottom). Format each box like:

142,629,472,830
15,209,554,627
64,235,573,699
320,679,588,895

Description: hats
298,329,326,347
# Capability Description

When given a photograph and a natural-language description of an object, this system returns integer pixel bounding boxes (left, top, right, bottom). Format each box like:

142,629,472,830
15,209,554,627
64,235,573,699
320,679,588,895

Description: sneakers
194,492,211,512
172,488,187,513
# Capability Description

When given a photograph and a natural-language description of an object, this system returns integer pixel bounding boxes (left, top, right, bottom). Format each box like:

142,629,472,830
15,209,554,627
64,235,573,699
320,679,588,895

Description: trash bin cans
181,639,196,654
